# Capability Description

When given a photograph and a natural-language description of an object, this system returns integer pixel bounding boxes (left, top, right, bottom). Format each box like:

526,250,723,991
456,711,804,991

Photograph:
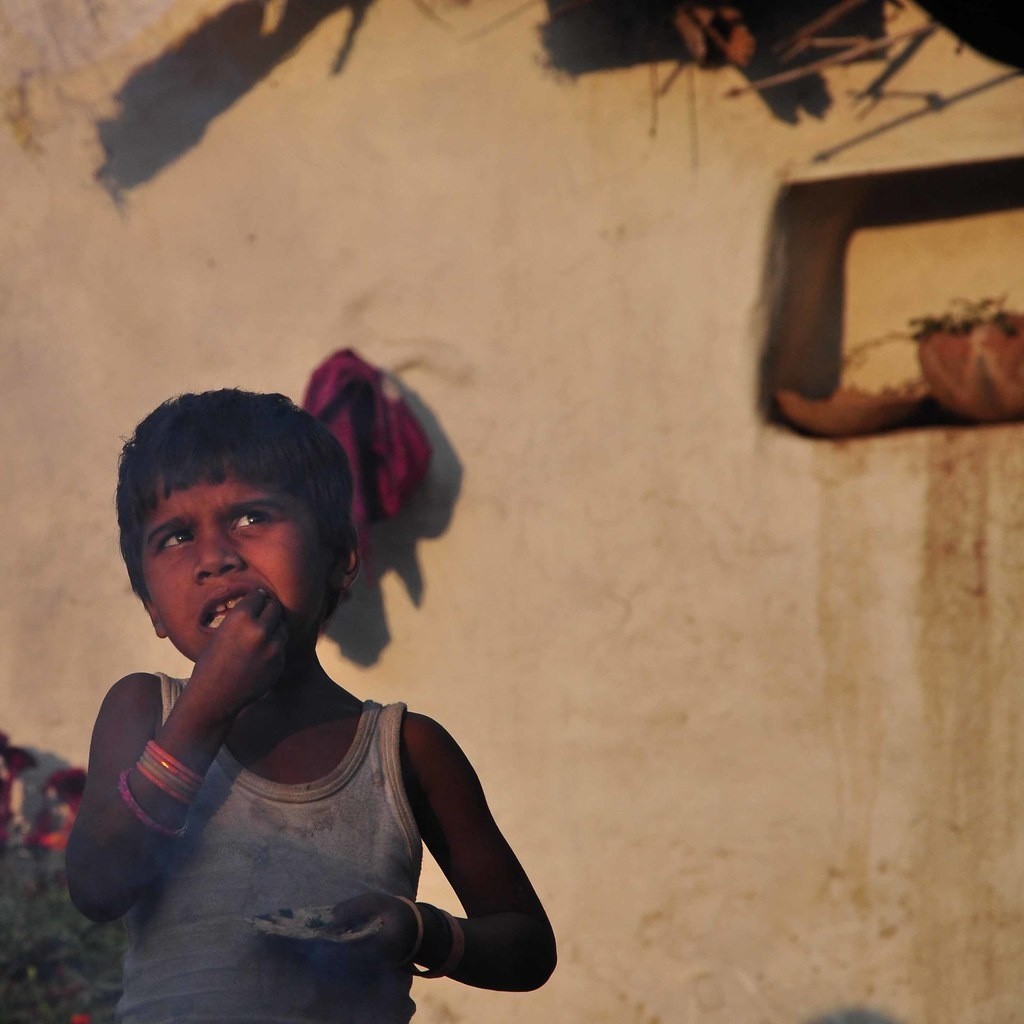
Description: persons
54,389,559,1024
0,732,87,850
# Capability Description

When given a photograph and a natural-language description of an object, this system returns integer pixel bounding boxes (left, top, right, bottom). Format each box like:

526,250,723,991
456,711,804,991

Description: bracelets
394,895,424,964
413,901,465,979
118,766,193,843
136,739,205,804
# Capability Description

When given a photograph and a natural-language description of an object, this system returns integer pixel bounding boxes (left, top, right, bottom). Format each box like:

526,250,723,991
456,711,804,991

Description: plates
246,905,382,943
